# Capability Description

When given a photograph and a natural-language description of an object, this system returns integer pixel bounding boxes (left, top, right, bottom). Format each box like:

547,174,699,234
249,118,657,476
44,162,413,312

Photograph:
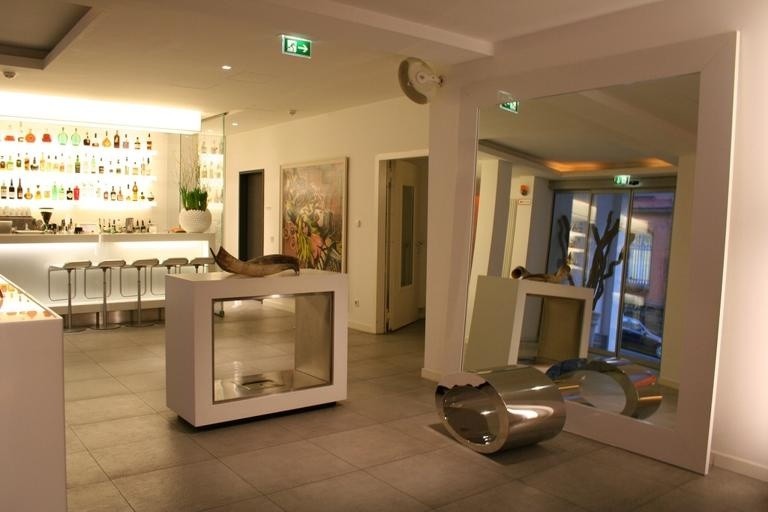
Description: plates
15,229,44,234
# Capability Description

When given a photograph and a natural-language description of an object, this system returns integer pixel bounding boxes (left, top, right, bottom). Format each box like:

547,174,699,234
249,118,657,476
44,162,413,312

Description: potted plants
177,161,212,233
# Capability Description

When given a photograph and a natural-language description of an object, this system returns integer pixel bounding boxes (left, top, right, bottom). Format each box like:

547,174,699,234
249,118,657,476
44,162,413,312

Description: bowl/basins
0,221,12,233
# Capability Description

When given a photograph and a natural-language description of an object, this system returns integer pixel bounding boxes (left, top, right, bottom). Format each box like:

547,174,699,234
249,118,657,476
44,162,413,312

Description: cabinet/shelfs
0,273,68,512
0,115,164,208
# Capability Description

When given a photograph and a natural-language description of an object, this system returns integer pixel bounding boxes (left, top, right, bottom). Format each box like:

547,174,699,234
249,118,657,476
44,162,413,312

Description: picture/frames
279,160,346,272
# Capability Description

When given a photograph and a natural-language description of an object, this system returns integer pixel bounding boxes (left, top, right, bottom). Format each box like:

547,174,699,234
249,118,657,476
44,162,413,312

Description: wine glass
39,208,54,231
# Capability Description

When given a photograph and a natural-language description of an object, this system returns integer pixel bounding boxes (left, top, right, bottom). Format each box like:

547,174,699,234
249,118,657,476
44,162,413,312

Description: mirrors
440,28,741,478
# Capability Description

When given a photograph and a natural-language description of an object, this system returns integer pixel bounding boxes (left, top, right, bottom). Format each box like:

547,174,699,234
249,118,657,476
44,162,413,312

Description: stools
48,257,217,333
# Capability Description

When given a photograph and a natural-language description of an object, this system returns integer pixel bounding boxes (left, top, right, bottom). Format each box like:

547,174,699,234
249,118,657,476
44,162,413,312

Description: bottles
52,218,75,233
1,123,156,203
0,206,31,217
99,218,152,233
0,282,31,304
199,138,224,205
